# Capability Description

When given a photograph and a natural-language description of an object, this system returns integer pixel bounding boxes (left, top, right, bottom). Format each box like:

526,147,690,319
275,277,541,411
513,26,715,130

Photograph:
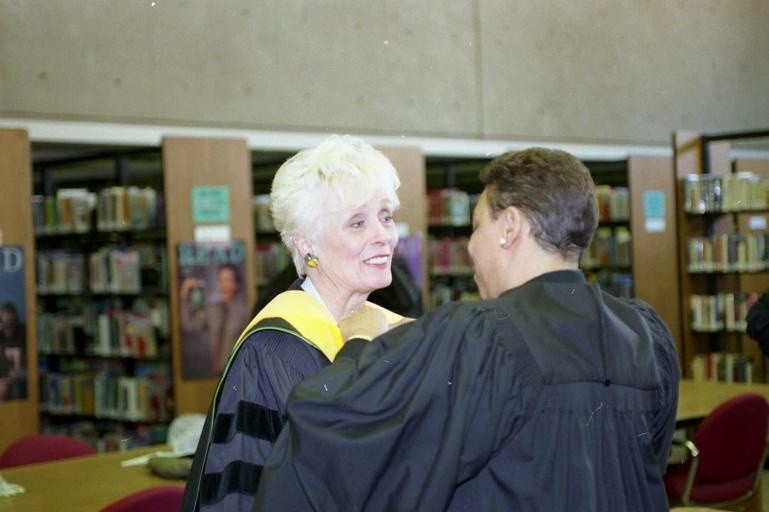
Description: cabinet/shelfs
426,156,680,347
673,130,769,384
0,129,38,437
256,146,432,318
33,137,257,453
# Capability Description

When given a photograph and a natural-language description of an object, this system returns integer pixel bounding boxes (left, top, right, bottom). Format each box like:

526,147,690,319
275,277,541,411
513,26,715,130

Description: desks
1,440,243,511
665,380,765,427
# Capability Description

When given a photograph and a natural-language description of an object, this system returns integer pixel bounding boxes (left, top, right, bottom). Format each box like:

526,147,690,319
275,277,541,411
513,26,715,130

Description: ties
215,304,230,354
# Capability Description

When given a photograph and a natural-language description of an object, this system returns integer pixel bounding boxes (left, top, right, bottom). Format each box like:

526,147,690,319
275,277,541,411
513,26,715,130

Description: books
256,240,290,288
429,236,475,267
691,291,764,332
38,358,173,421
428,189,481,228
254,193,273,231
690,342,758,383
36,243,165,295
430,278,481,310
586,271,634,298
36,296,169,358
580,227,634,268
41,423,169,450
685,172,769,212
30,186,165,233
595,185,631,221
686,212,769,274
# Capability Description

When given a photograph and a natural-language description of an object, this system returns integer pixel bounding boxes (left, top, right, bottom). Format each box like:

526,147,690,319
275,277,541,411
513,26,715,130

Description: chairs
663,393,768,510
0,435,94,469
102,486,191,511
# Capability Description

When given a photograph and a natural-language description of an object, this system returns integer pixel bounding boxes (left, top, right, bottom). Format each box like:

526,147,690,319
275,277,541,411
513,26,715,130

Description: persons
182,134,422,512
248,149,680,512
181,262,248,374
0,302,26,400
